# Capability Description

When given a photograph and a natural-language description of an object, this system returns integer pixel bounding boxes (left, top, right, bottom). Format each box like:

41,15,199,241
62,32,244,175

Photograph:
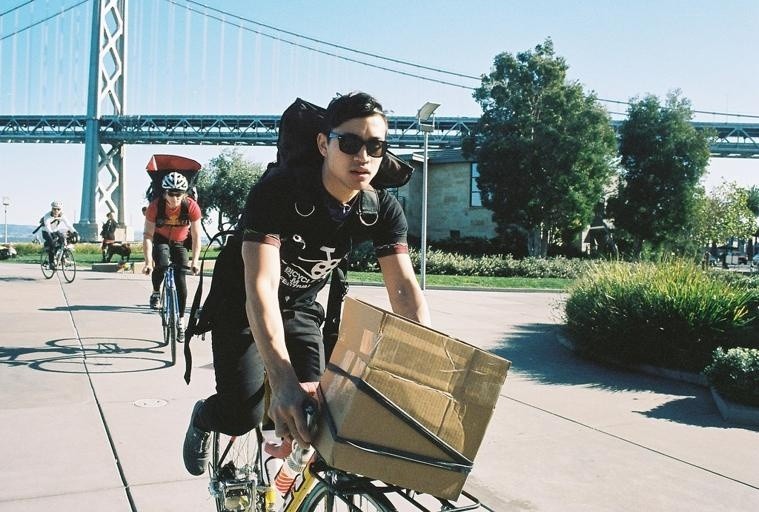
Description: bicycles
147,261,185,370
41,231,79,283
203,378,479,512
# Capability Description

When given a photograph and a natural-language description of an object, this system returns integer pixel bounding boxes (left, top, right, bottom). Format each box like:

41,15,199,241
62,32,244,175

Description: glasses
329,132,391,158
168,192,181,196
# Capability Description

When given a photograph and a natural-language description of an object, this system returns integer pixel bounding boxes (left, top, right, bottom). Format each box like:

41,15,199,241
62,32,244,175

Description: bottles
274,442,311,498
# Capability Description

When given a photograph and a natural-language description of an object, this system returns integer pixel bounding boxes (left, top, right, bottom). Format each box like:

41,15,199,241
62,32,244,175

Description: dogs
104,241,132,263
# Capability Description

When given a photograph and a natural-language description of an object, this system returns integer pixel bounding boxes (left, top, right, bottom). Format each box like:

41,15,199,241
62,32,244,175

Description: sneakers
183,400,211,475
177,325,185,342
150,294,161,309
49,263,56,270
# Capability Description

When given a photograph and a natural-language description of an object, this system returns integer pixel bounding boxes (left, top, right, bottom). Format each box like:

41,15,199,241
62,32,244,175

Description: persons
42,199,77,271
182,90,431,476
99,212,117,262
144,170,203,343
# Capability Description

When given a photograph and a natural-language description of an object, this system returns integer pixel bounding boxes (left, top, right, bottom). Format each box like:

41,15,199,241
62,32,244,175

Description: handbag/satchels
102,232,109,238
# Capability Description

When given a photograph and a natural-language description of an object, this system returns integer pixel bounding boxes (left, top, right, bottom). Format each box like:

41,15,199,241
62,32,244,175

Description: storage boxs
146,155,201,204
307,292,512,506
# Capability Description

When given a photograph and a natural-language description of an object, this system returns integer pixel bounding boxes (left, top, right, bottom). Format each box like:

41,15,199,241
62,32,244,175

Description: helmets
51,201,63,209
162,171,188,191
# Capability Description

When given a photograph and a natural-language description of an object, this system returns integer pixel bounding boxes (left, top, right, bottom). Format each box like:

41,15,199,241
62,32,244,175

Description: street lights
2,195,10,244
410,97,440,287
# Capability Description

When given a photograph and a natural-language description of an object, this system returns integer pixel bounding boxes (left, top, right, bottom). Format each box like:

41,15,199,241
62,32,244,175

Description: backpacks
265,98,414,228
146,154,201,226
